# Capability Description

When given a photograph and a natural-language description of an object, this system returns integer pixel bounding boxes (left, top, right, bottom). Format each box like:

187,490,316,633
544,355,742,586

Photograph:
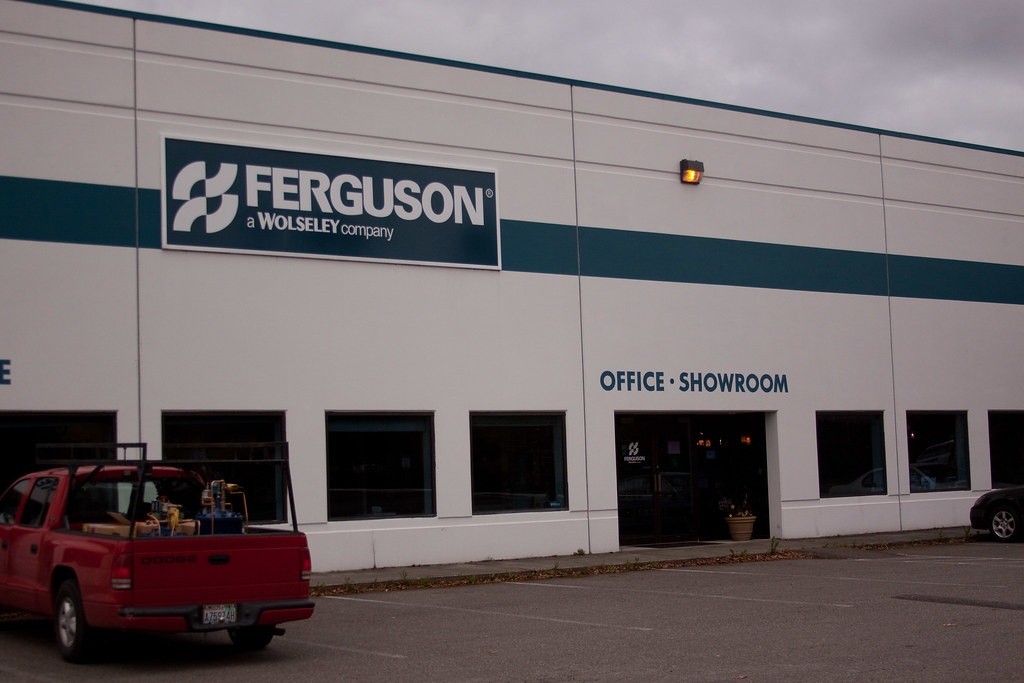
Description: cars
970,484,1024,541
830,465,971,497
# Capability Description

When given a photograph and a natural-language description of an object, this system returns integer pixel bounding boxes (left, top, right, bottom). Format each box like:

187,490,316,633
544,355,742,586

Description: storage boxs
81,512,201,537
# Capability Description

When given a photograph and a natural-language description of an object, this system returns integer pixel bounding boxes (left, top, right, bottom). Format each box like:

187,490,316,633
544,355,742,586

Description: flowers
719,493,756,517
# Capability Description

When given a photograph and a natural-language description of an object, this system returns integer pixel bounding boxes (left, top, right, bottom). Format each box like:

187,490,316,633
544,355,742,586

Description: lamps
680,159,705,186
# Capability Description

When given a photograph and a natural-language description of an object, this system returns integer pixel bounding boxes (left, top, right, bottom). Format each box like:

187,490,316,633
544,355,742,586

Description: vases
725,517,757,541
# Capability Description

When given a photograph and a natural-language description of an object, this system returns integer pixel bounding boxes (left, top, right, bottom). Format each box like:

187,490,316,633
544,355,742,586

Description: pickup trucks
0,441,316,662
